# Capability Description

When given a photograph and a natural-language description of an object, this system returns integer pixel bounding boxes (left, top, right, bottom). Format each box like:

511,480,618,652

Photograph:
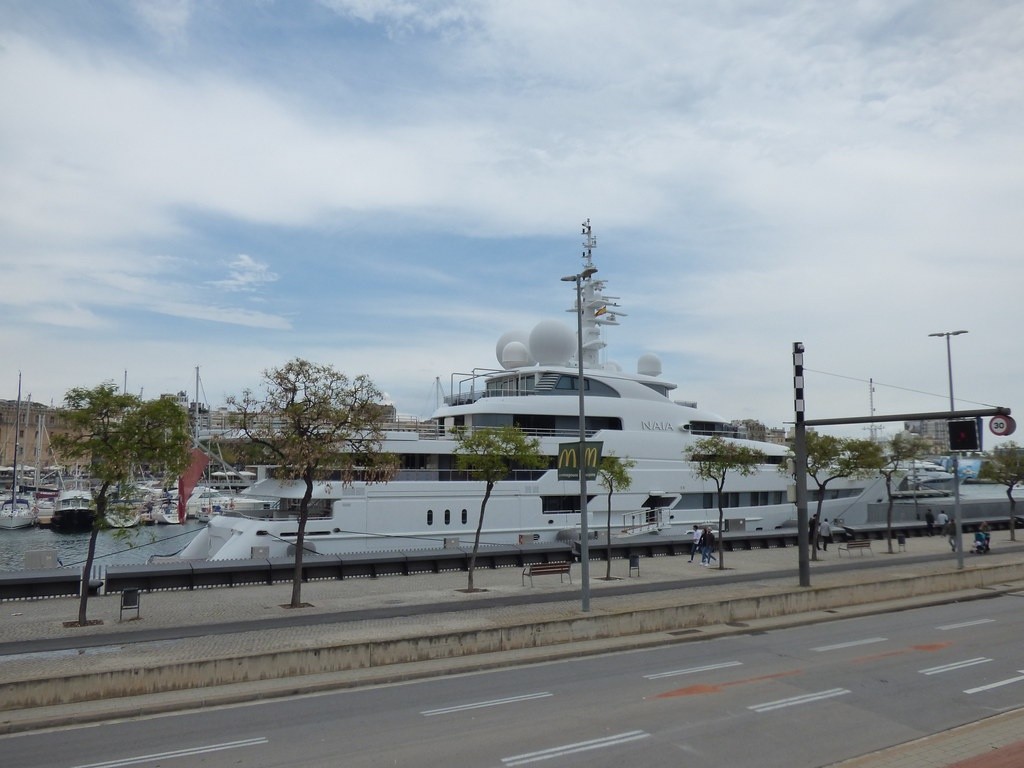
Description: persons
203,498,236,512
808,514,822,550
820,518,832,550
948,520,956,552
925,509,935,536
937,510,948,537
687,525,719,566
971,521,991,554
733,424,746,439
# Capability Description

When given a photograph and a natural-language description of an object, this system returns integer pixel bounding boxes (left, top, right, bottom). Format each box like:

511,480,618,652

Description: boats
831,378,967,496
176,218,910,559
1006,481,1024,489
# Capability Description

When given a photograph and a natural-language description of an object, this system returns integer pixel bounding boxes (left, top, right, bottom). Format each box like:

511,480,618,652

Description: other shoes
688,560,693,562
700,562,703,566
705,564,710,567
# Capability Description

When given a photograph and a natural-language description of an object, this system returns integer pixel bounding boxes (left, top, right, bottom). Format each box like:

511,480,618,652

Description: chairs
629,553,640,578
120,588,140,620
897,533,905,552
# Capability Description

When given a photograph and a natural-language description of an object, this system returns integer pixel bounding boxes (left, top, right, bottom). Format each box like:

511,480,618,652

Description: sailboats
0,364,276,532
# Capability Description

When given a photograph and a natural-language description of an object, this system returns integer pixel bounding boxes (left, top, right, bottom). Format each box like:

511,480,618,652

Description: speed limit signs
989,415,1011,436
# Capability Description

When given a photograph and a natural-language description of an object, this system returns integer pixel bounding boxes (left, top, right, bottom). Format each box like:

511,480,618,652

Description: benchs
522,562,571,588
837,539,874,557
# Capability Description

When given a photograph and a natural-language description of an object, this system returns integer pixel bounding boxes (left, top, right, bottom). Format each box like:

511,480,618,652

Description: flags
595,307,607,316
178,447,212,524
